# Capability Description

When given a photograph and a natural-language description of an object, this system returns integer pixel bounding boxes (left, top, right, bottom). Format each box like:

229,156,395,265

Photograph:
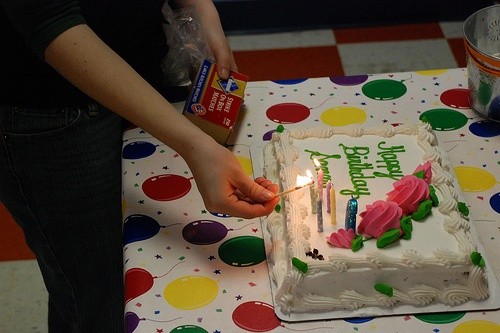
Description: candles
330,183,336,226
314,158,324,202
306,169,318,213
345,194,356,235
316,193,324,233
326,179,332,214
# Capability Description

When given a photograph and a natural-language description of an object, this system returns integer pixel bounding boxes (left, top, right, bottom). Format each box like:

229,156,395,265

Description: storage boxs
180,57,248,148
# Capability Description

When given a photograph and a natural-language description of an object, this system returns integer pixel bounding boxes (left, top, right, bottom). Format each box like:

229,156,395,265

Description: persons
0,0,279,333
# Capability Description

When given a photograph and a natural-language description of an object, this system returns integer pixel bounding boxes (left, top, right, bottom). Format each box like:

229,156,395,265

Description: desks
122,67,500,333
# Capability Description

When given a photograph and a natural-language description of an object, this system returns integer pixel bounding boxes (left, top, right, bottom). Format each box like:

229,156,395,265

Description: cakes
262,121,490,315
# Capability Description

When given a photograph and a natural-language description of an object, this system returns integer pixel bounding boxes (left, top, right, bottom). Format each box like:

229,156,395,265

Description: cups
462,4,500,122
